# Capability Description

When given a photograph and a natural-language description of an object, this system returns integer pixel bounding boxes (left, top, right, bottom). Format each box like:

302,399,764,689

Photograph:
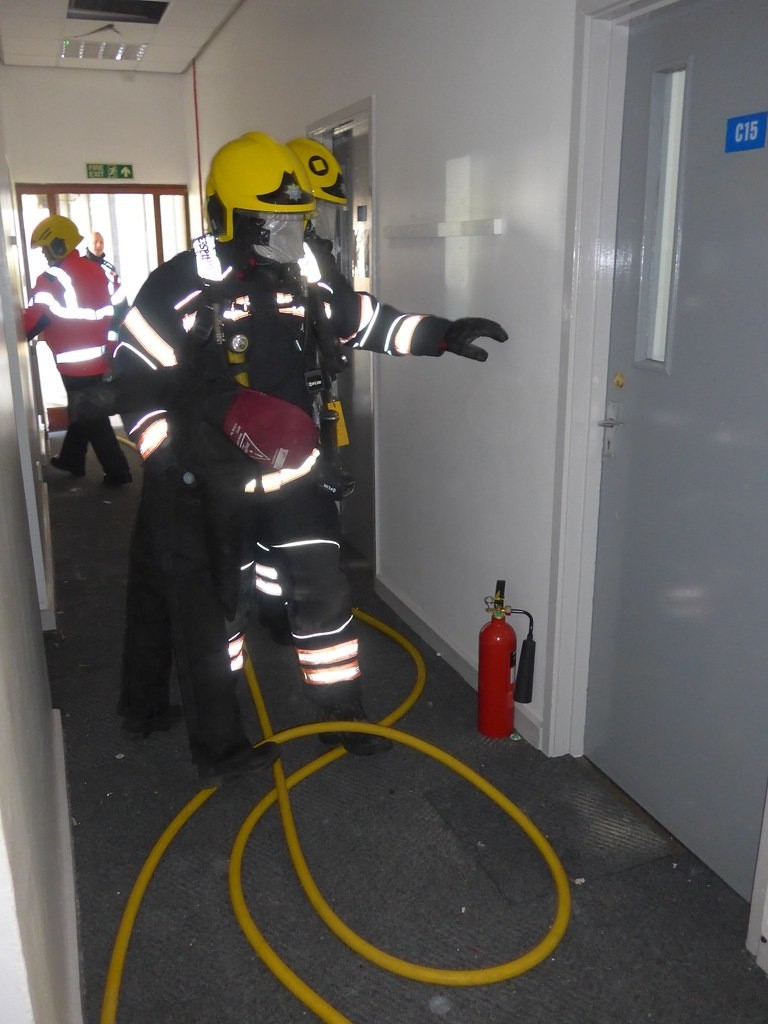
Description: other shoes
196,741,281,789
49,456,85,476
122,705,169,737
270,628,292,645
319,700,394,759
102,473,133,487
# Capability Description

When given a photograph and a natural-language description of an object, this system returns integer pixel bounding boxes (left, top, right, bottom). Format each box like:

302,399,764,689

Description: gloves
148,440,205,508
445,317,507,362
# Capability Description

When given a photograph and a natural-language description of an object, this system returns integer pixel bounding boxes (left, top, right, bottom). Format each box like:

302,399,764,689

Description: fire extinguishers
478,580,537,736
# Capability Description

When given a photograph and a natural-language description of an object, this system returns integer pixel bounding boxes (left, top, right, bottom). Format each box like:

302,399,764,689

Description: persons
110,131,510,779
22,215,134,488
81,232,120,297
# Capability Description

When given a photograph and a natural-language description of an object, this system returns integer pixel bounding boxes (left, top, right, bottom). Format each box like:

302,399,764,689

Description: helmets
287,137,350,206
30,215,83,258
203,132,320,242
